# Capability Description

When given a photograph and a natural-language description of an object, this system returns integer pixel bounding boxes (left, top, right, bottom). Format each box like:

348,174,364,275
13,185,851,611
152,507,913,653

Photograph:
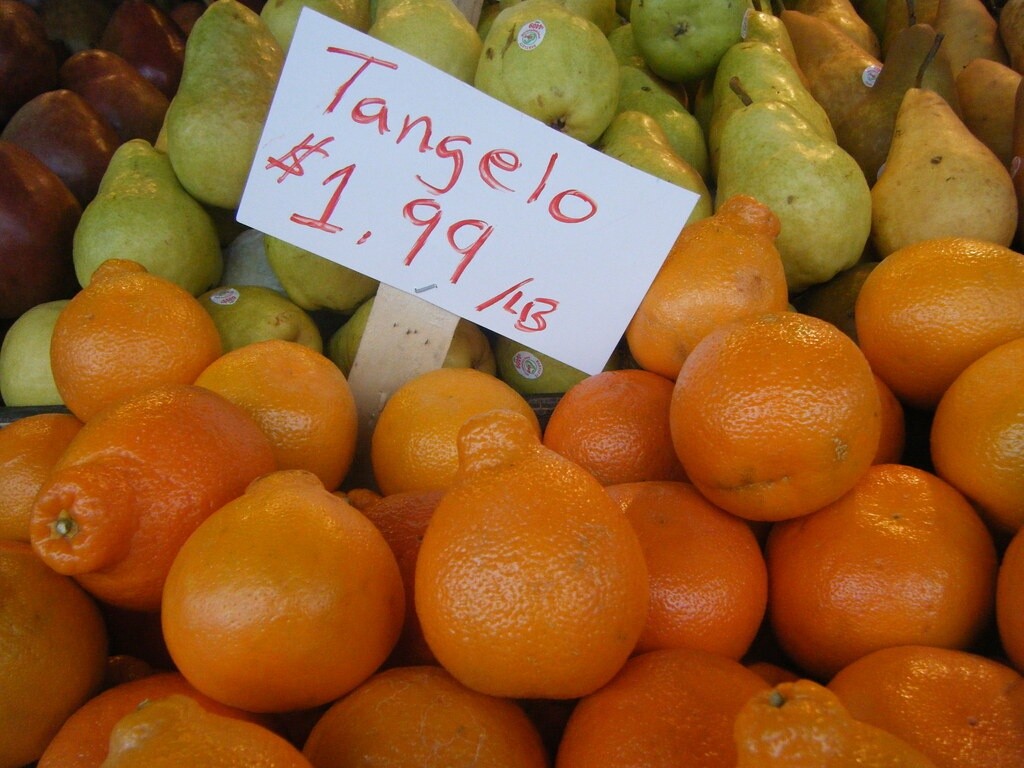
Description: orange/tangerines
0,194,1024,768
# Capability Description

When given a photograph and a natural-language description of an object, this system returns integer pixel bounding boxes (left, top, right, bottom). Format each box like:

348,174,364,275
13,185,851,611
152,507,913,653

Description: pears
1,0,1024,408
